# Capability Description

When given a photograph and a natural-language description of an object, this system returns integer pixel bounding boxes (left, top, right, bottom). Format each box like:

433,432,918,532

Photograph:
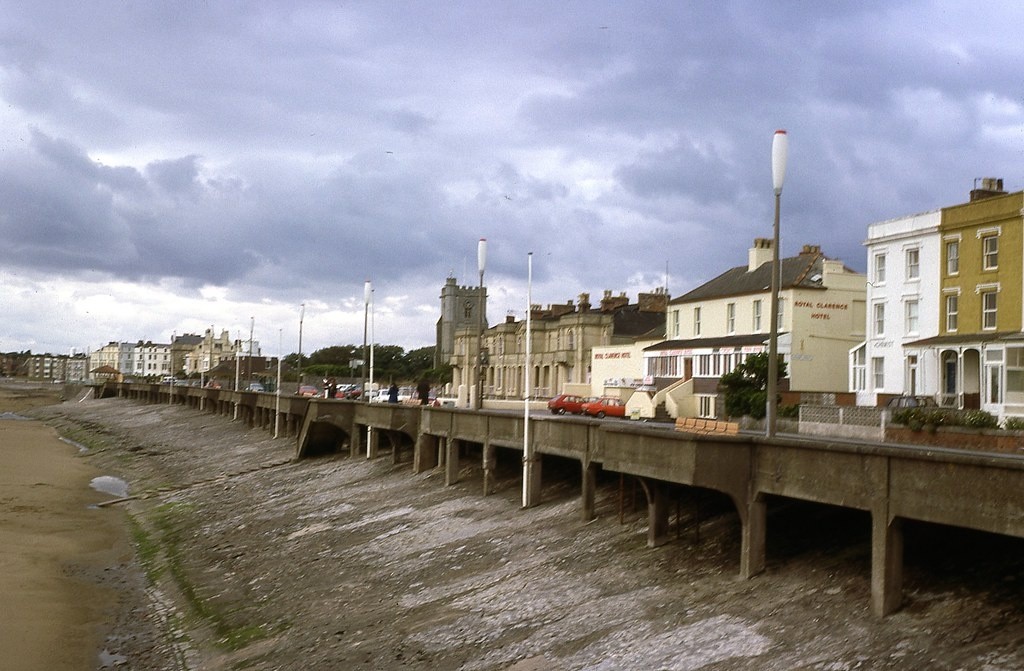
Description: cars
581,397,626,419
547,395,587,416
372,388,404,403
246,383,265,392
882,396,939,409
161,376,222,388
296,383,379,401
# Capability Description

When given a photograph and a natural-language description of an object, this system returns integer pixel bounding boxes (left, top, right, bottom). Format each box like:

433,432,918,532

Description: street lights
248,317,255,391
476,237,487,408
362,278,372,401
298,303,306,394
766,128,788,436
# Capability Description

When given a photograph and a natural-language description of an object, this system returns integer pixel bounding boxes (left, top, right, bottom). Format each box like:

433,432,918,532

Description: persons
387,383,399,403
416,375,431,406
